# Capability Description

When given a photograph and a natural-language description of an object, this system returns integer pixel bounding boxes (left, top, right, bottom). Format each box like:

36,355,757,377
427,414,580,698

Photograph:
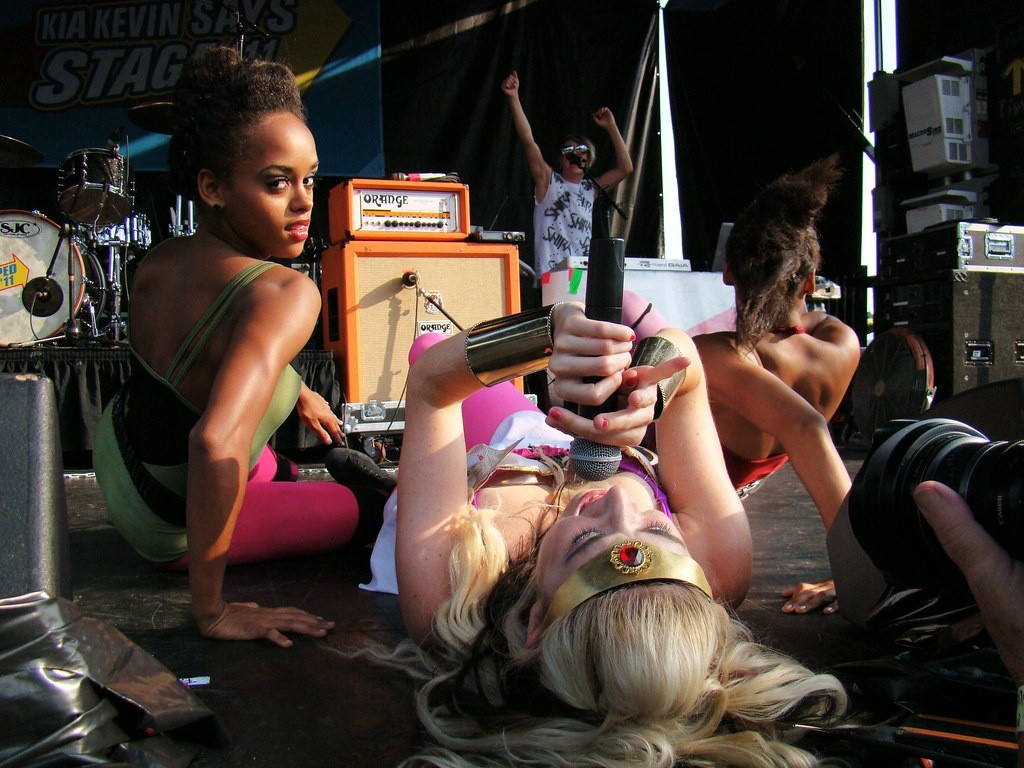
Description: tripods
8,176,130,349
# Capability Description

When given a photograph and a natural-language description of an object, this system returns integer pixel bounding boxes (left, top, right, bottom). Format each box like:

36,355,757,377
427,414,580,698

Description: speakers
873,268,1024,407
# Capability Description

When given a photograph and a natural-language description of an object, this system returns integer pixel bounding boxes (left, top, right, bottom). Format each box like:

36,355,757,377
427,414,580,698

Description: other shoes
327,449,398,493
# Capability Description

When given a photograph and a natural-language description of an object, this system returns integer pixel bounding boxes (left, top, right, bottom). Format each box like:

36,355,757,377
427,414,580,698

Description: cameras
848,419,1024,586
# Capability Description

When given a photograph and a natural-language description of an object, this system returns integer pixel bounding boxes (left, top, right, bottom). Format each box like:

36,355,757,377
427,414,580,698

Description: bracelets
629,336,687,423
464,300,561,389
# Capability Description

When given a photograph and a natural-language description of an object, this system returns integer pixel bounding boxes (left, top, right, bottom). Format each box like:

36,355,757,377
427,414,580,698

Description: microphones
34,291,51,302
107,127,123,147
566,152,588,163
570,198,624,481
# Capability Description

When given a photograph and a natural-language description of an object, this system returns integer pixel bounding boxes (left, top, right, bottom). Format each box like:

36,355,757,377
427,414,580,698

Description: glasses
562,145,589,155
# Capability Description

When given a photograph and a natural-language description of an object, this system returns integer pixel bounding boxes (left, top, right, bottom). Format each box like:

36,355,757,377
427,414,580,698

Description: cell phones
896,711,1018,755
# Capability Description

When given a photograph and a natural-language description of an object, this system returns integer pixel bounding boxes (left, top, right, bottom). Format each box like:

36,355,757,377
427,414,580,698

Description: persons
502,70,635,312
90,45,359,648
638,157,856,617
324,291,852,768
915,480,1023,698
